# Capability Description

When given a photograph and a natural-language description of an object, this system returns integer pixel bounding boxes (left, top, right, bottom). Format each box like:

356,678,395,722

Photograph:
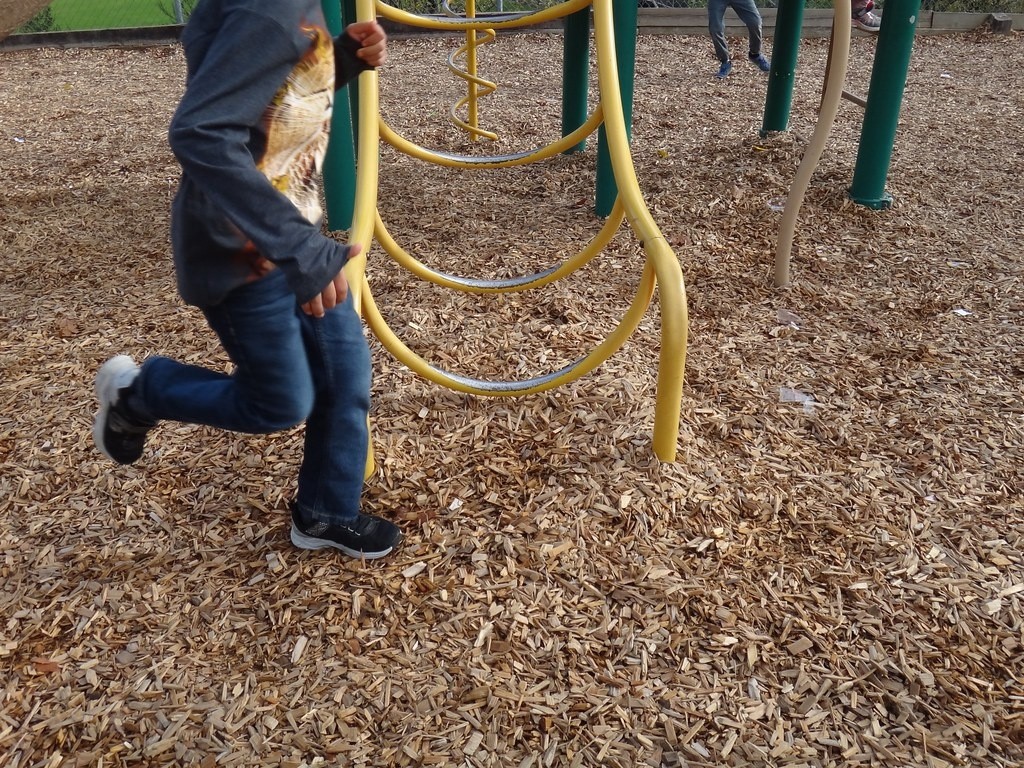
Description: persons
707,0,771,80
89,0,402,560
851,0,882,31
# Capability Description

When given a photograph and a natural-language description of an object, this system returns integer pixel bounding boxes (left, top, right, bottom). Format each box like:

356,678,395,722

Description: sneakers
287,499,404,559
851,0,881,31
716,60,733,79
92,355,160,465
748,51,771,71
